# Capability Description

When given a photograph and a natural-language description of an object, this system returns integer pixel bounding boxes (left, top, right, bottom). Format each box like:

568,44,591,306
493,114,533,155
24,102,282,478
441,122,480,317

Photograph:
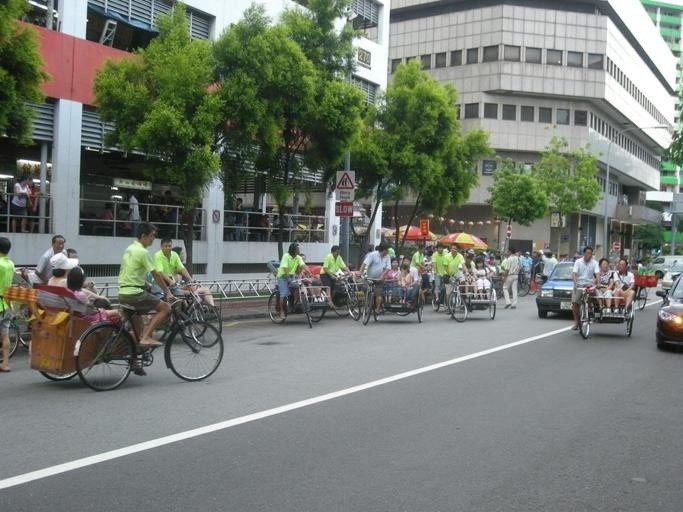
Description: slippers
139,340,163,347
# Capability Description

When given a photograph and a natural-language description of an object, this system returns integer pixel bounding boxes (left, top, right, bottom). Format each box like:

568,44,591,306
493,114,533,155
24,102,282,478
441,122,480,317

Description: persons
9,175,32,233
593,258,613,316
0,223,201,378
356,236,582,315
607,259,635,314
318,245,352,309
569,246,600,331
275,243,313,320
24,179,38,233
295,254,325,303
98,186,293,241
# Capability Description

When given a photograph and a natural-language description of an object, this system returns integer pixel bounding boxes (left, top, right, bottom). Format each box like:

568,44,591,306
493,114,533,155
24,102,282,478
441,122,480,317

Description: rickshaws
0,267,47,362
573,283,635,339
267,255,545,329
126,280,223,349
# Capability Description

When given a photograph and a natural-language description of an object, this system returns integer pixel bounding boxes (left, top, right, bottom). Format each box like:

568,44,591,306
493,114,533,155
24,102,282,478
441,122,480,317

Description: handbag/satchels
622,284,629,291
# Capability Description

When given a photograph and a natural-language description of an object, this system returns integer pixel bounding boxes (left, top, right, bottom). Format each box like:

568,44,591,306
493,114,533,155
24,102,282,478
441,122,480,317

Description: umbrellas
436,231,487,258
388,223,436,249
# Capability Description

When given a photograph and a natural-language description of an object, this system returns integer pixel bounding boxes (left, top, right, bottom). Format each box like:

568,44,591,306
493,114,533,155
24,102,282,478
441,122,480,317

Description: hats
49,253,79,270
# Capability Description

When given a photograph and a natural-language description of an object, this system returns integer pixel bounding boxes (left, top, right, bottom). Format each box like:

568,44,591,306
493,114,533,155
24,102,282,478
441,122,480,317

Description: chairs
34,283,103,323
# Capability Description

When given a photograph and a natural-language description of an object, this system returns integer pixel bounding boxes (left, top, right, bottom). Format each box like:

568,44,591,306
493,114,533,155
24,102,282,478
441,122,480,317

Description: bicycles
631,284,648,310
73,292,223,391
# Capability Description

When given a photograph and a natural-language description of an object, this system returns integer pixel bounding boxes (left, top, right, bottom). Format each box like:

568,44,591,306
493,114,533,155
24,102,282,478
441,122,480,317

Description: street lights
602,124,668,260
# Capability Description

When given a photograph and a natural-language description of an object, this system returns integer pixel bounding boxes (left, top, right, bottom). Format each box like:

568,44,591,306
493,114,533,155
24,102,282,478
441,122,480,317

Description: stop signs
612,241,620,252
506,224,512,240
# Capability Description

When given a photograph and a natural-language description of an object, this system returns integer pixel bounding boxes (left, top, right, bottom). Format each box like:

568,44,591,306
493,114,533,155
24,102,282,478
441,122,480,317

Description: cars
656,260,682,303
654,272,683,350
536,262,577,318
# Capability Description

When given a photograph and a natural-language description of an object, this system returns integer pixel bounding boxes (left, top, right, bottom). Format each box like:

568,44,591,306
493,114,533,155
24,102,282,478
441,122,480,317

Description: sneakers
602,308,628,314
314,298,318,303
318,298,323,303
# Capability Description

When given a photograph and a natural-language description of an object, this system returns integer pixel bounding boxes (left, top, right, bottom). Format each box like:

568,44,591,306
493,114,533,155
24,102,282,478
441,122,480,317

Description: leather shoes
279,311,286,319
